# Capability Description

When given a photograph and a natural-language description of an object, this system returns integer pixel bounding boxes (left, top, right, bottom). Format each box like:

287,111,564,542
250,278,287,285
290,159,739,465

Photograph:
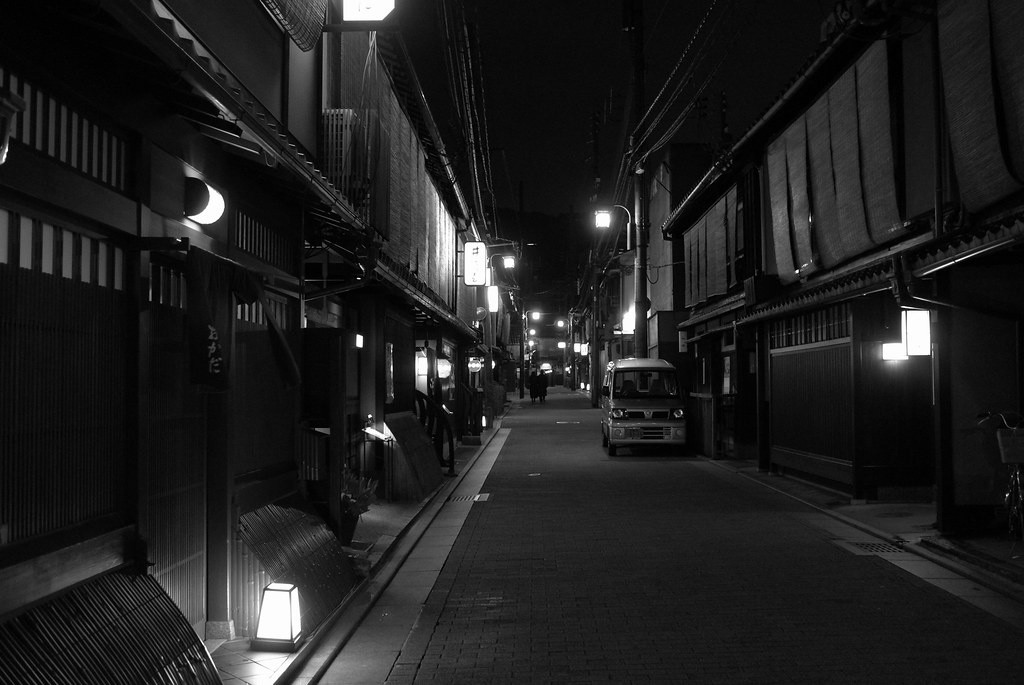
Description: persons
537,370,549,403
529,371,538,402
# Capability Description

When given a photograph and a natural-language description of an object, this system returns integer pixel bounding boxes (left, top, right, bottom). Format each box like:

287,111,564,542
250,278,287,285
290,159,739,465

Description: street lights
593,202,651,391
557,317,576,391
484,252,518,419
520,309,540,399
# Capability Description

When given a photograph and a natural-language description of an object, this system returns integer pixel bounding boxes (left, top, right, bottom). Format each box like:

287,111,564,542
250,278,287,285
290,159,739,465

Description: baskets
996,428,1024,464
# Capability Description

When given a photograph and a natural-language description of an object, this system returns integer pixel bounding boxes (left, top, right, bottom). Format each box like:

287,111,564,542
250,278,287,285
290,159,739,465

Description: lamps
324,0,398,32
251,582,303,653
882,343,909,360
457,240,486,286
901,309,933,356
185,177,226,224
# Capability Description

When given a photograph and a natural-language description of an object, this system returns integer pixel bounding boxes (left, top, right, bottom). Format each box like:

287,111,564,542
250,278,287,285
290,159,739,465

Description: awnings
97,0,365,236
304,253,478,345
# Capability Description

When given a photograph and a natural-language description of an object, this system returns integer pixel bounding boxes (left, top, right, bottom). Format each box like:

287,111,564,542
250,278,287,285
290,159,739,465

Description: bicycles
529,383,536,403
975,409,1024,561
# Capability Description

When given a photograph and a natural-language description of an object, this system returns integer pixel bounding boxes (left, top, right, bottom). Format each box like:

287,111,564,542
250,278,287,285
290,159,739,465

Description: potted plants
340,468,376,545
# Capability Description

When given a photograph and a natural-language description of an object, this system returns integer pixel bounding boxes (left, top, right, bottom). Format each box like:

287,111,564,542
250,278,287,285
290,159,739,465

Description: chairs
621,380,642,400
649,379,668,400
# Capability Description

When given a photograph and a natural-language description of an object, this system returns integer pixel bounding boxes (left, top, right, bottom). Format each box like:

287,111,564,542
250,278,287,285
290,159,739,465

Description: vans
600,357,691,457
